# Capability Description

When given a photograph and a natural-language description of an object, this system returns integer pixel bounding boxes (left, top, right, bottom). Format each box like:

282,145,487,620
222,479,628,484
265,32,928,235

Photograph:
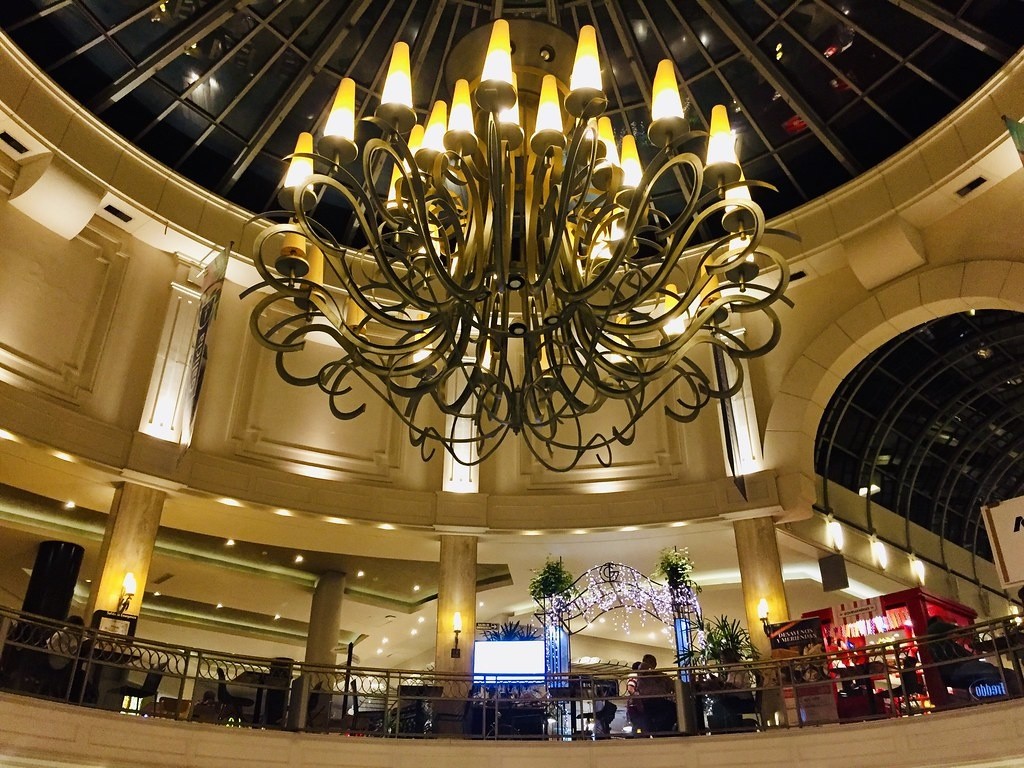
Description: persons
400,686,542,738
712,648,755,734
594,687,611,740
928,616,1024,700
43,616,86,701
626,654,676,737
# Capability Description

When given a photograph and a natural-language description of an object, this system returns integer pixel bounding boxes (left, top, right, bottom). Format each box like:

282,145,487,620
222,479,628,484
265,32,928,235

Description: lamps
450,611,461,658
869,533,888,571
826,513,844,553
909,552,926,587
118,572,136,613
244,17,809,473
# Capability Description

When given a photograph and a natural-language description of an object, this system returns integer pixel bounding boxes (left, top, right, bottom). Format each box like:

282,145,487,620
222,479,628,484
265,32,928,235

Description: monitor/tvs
473,639,546,685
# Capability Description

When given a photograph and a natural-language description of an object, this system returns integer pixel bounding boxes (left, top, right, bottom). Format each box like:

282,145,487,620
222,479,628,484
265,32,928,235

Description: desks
548,686,610,740
682,679,724,736
230,671,293,729
968,631,1024,666
397,685,445,739
831,660,900,720
76,645,140,704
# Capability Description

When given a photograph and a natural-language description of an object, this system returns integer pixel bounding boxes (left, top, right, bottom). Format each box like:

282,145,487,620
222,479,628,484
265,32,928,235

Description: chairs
729,671,767,732
351,679,385,736
504,687,545,741
627,696,677,738
102,662,167,718
876,656,923,717
435,690,474,739
217,668,255,728
928,644,1001,706
287,681,323,727
